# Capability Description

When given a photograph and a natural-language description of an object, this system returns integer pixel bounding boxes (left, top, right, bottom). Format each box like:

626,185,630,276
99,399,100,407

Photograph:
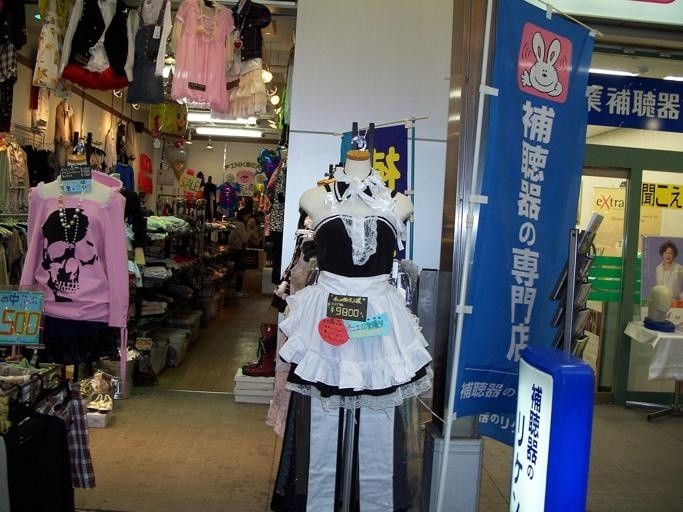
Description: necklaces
61,198,80,248
56,183,82,228
232,2,251,52
199,0,218,43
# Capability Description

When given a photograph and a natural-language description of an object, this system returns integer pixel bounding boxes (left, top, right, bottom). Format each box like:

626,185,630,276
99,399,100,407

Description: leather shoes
87,394,113,411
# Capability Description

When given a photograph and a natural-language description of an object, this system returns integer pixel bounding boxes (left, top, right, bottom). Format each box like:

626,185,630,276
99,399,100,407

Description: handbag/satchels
244,250,259,269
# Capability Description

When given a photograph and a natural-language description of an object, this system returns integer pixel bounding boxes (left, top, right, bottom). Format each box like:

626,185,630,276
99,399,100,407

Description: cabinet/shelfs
131,228,238,339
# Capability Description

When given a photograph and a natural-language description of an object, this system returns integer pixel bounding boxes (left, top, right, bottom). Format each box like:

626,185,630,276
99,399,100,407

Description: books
548,214,605,355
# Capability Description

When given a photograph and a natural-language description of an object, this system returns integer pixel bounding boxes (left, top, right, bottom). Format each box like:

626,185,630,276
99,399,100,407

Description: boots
243,323,277,377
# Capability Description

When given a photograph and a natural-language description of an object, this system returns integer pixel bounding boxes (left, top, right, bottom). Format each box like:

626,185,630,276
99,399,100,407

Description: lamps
262,62,280,106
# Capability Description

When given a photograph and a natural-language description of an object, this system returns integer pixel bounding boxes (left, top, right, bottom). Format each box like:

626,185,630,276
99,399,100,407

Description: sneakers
237,291,248,298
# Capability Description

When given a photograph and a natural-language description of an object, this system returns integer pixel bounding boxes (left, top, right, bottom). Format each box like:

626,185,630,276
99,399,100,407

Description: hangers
5,374,71,424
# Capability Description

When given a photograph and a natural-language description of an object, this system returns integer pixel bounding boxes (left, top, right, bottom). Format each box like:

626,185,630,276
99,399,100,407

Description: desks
622,319,683,422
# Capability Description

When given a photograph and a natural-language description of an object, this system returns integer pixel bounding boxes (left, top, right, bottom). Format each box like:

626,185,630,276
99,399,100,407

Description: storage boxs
154,310,204,368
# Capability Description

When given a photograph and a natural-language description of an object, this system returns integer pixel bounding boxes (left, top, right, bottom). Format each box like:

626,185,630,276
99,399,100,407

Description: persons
15,152,131,363
246,211,264,250
656,239,683,301
275,147,436,411
226,208,255,297
